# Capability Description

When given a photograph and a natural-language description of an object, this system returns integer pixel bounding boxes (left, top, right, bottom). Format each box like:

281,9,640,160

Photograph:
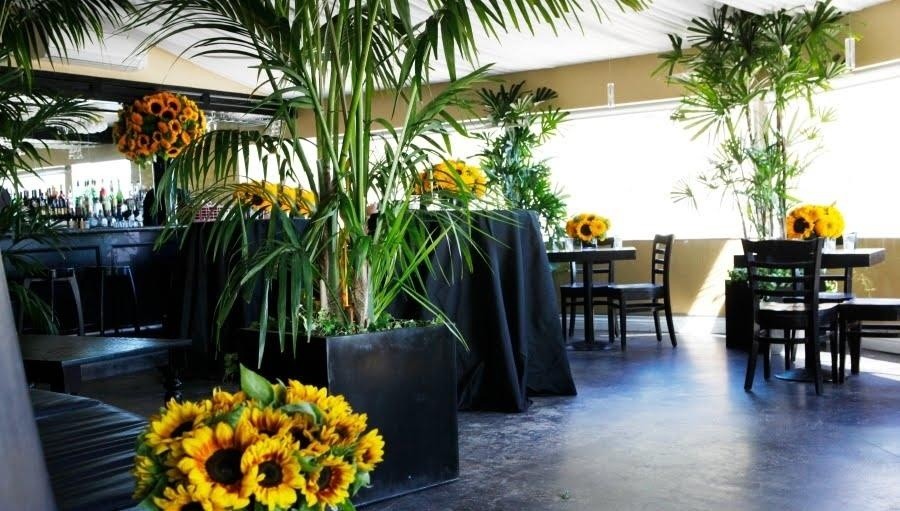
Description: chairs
733,237,900,394
543,235,676,350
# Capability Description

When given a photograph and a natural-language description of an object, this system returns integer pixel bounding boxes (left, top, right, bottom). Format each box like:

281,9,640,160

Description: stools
82,264,142,336
15,268,85,337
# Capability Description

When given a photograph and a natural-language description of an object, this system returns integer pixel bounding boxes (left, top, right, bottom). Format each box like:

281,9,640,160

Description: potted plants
115,0,646,508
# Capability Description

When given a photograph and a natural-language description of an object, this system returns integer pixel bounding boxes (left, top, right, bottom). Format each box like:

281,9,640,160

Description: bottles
11,177,147,229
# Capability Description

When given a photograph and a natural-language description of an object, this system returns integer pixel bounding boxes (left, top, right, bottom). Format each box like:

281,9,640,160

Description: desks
20,332,193,410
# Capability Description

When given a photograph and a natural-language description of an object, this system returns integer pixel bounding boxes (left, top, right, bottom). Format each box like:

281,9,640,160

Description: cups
825,237,835,252
564,238,574,251
843,234,854,252
613,235,622,249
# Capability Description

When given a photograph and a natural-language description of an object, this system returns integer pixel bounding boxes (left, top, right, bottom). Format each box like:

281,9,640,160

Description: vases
804,233,825,248
153,156,172,225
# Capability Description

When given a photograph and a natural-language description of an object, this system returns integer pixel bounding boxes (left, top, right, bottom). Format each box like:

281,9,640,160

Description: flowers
785,205,845,241
232,180,315,217
566,214,609,241
408,160,489,200
112,91,206,168
128,363,386,511
